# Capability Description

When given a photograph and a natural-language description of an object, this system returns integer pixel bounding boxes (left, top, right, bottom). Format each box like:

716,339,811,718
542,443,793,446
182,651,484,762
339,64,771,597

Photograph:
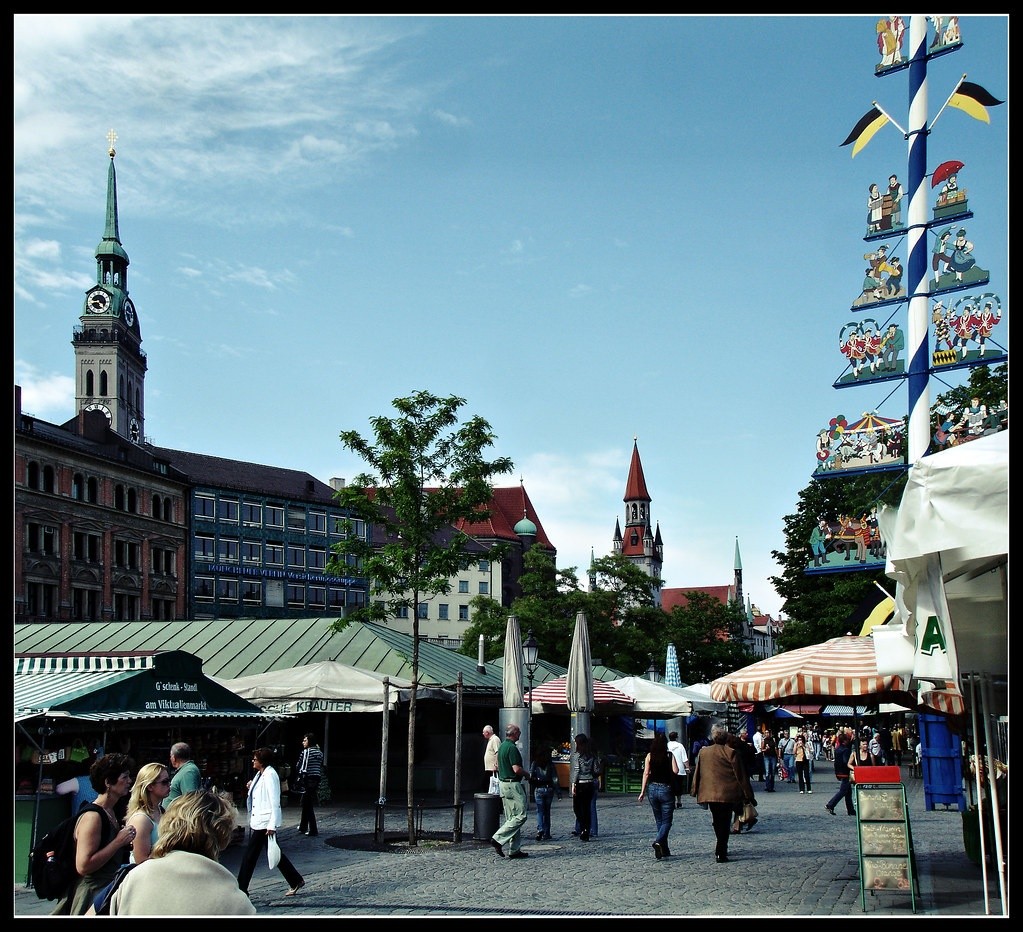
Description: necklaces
103,808,117,822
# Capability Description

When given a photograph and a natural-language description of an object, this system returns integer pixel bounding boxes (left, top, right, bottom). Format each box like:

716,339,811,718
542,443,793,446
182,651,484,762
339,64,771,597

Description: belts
499,778,517,782
579,780,593,783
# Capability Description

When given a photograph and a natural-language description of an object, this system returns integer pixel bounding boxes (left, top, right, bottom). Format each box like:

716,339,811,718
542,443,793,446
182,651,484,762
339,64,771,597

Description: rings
132,832,135,835
129,831,132,834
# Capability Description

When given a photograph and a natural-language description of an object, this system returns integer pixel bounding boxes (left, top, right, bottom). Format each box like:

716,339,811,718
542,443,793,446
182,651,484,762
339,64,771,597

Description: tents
604,676,728,794
222,661,456,712
14,648,299,798
884,428,1008,915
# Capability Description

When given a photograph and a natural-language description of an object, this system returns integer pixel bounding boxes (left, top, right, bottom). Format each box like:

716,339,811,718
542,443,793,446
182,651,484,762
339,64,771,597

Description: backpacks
32,802,109,902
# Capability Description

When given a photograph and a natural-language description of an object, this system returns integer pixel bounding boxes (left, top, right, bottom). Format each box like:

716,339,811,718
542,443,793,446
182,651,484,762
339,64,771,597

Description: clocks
83,403,112,427
87,290,110,313
124,300,135,328
129,418,140,445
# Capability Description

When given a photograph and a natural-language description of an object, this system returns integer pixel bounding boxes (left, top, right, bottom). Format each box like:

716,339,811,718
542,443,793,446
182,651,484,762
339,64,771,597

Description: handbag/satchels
780,747,786,759
849,770,856,783
290,770,307,793
738,802,759,822
488,771,500,795
781,765,789,778
268,832,281,869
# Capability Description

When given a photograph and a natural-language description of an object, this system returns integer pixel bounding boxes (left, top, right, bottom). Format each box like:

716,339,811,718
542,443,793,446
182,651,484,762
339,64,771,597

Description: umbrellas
710,630,964,766
566,609,596,712
665,643,683,687
501,615,526,708
523,673,637,707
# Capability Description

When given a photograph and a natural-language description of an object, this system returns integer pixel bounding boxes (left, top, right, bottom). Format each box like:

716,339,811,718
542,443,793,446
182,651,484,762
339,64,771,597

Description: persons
490,723,532,859
237,748,306,899
295,733,322,836
162,742,201,810
481,725,505,813
48,754,137,916
84,787,256,915
638,731,680,859
666,732,691,808
735,721,906,817
119,764,171,864
570,735,603,840
528,745,562,842
692,724,758,861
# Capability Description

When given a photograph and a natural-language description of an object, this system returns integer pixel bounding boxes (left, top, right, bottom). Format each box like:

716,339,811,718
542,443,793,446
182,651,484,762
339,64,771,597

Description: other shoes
746,819,758,831
305,832,318,835
491,839,505,857
509,851,528,858
824,805,836,815
653,842,672,859
716,856,729,863
535,831,552,841
729,828,740,834
848,810,856,815
285,879,305,896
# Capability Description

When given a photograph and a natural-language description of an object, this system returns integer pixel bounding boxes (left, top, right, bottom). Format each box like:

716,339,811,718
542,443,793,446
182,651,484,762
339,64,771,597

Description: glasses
153,778,170,786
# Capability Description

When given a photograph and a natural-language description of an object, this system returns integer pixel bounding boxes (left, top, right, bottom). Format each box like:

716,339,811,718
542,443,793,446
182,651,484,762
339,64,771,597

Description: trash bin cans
474,792,502,840
